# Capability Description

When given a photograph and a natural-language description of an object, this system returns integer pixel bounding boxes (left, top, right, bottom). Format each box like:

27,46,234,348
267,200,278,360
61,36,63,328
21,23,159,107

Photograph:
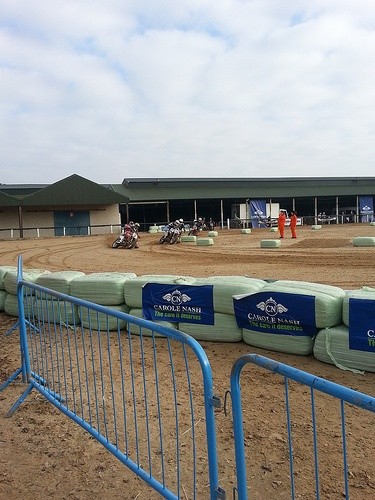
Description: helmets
128,221,134,227
198,218,202,223
179,218,183,223
135,223,140,229
175,220,180,225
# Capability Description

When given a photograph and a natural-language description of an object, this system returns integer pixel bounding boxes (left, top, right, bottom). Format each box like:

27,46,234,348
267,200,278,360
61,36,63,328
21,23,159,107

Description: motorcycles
187,222,201,236
111,226,138,249
159,226,180,245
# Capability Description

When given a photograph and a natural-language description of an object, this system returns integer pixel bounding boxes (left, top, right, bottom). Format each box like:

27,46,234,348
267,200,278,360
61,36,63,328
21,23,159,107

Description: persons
278,211,287,238
122,214,216,249
290,210,298,238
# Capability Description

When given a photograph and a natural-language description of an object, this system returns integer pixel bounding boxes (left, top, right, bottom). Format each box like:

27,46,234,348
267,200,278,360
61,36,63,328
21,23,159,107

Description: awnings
0,172,131,238
